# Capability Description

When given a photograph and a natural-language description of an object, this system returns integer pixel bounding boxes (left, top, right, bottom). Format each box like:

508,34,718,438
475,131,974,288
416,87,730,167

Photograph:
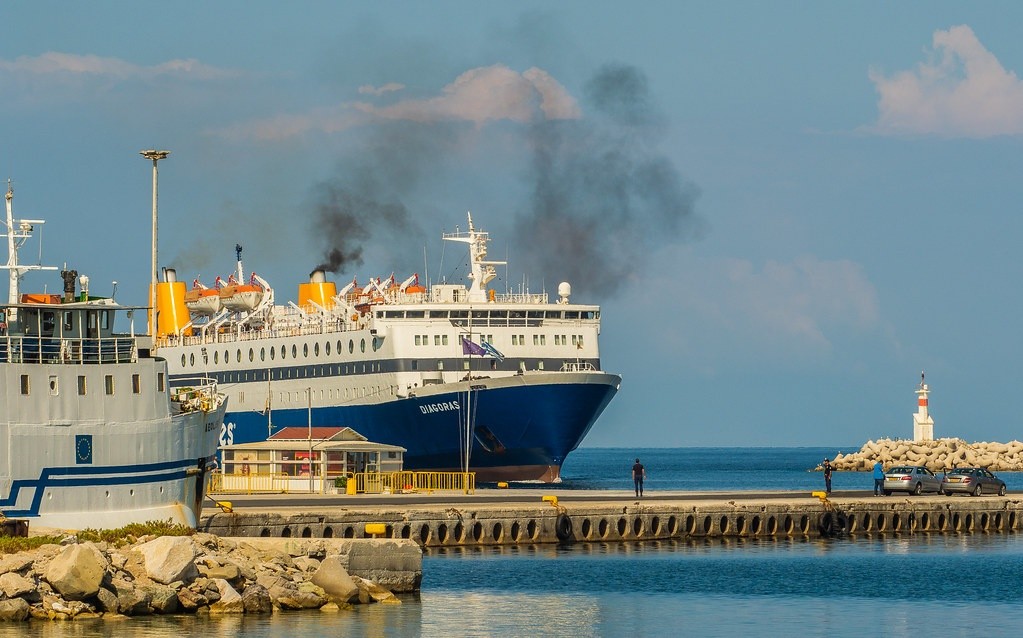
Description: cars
883,466,945,497
943,466,1006,497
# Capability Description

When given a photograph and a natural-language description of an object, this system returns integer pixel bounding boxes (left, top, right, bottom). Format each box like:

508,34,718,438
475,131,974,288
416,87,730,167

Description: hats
824,458,829,461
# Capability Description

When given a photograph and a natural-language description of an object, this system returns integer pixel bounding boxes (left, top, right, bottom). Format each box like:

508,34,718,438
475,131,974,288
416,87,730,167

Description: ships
143,211,623,482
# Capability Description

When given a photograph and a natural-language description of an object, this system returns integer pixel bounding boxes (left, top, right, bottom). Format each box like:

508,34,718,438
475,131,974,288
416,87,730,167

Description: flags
462,337,486,357
479,338,505,363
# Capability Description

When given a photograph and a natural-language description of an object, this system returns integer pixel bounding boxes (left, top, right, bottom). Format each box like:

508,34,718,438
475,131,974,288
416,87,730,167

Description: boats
219,284,263,313
183,289,221,313
0,176,229,540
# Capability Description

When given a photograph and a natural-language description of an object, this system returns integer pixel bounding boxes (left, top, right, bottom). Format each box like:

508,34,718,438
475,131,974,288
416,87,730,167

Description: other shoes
826,492,830,496
874,494,879,496
881,494,886,496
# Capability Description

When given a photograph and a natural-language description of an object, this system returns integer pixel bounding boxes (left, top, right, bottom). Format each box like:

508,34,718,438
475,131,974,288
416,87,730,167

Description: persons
823,458,832,496
632,459,647,497
874,460,886,496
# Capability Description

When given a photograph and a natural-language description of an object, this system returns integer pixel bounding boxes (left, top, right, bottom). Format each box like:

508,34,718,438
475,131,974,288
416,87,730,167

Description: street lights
137,149,172,355
